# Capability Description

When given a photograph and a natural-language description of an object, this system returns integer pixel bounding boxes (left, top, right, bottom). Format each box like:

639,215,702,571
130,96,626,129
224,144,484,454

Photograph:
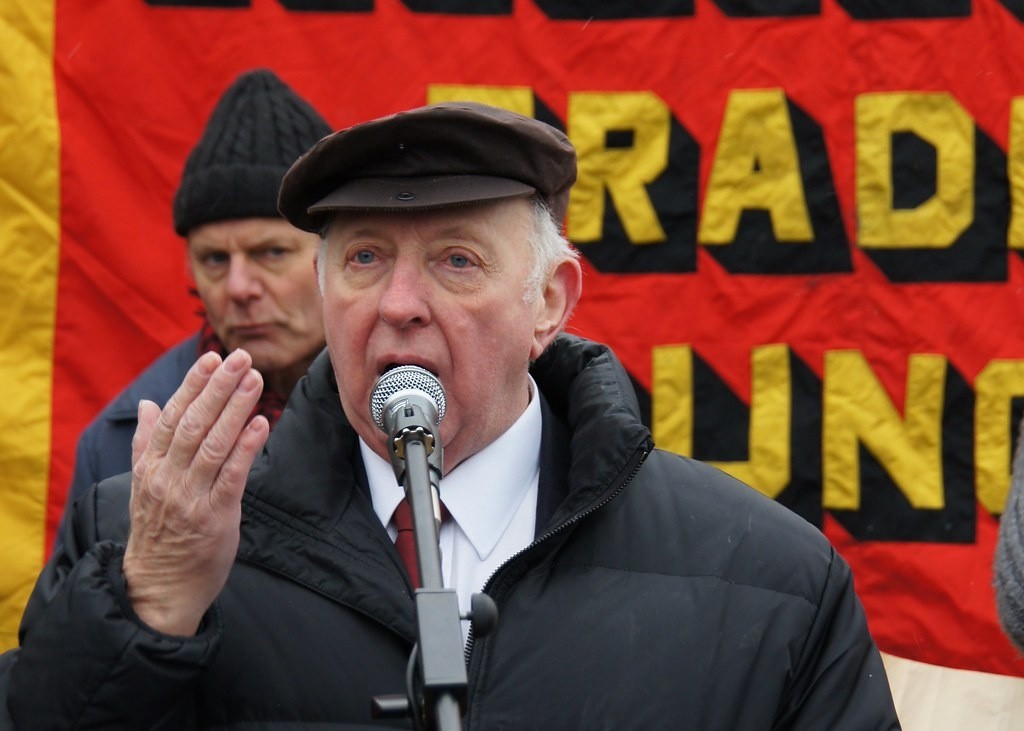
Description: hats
173,69,335,238
278,102,578,240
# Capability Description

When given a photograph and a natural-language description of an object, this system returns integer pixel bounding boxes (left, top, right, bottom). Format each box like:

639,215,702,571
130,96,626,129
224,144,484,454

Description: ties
390,496,451,590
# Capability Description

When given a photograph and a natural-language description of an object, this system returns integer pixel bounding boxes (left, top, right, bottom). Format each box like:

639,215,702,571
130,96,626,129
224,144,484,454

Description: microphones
369,366,447,546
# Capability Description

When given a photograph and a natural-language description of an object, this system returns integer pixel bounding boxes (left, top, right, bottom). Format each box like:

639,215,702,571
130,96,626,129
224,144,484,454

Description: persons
0,100,902,731
51,67,340,543
